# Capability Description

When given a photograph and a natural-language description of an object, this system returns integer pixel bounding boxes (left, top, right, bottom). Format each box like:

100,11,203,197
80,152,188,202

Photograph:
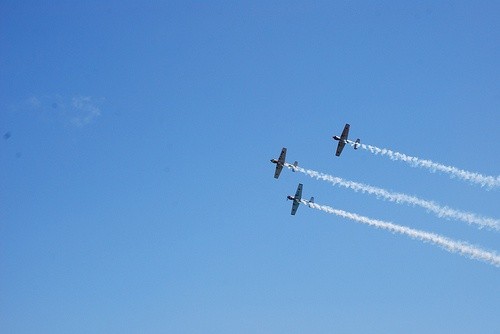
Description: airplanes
287,182,315,216
331,124,361,156
269,147,298,179
